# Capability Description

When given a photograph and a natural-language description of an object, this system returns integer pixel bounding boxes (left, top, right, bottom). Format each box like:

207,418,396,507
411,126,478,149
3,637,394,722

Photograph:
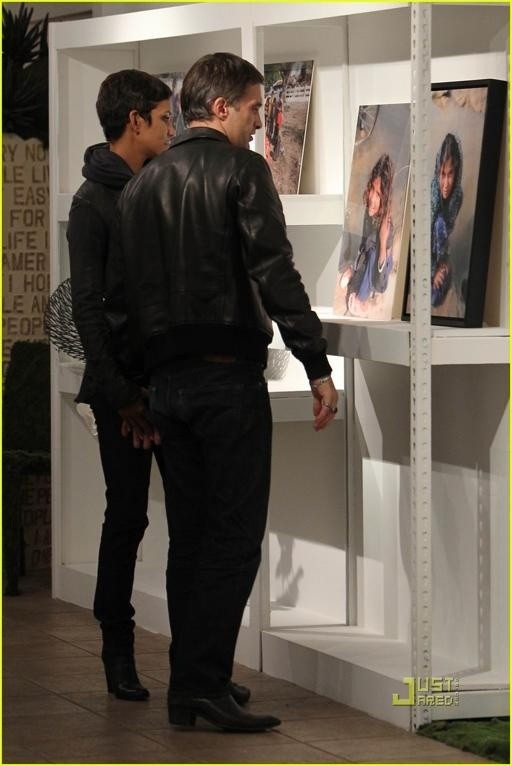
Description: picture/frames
401,79,507,329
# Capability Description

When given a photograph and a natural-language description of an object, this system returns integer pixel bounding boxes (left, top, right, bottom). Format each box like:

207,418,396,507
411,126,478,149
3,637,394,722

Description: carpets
418,719,511,765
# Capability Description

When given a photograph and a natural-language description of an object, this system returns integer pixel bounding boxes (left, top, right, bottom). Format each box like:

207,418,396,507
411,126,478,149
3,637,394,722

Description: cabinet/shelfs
48,4,511,740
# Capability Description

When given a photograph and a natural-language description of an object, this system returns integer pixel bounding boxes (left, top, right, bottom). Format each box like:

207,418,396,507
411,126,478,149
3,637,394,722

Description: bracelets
319,375,330,384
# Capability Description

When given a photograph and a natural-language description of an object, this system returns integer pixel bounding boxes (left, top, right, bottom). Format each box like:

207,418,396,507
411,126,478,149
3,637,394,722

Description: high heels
102,641,149,702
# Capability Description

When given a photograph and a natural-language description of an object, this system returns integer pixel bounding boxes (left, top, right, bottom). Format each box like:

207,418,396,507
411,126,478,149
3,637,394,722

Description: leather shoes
167,680,281,734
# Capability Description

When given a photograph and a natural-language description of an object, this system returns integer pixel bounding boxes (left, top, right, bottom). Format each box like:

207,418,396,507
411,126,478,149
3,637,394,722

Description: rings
322,401,330,408
331,407,337,413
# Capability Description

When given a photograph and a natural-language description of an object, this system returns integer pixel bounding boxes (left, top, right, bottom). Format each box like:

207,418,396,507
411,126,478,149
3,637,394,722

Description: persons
65,70,251,705
265,95,283,163
105,52,339,732
430,133,464,311
338,153,394,316
